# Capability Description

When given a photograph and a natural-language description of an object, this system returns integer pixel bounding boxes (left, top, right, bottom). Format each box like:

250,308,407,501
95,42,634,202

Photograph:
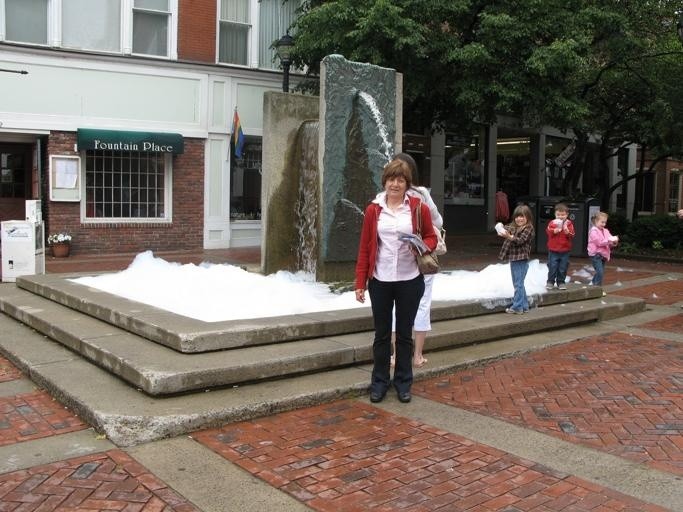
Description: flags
233,109,245,159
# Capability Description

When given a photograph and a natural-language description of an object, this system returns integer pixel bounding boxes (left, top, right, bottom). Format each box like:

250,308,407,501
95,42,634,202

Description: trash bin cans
517,196,601,258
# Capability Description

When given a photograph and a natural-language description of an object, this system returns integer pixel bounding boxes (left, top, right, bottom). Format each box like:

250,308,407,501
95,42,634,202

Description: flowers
45,232,72,245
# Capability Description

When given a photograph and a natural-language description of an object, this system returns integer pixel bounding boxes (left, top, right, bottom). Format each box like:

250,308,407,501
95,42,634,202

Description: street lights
277,28,300,93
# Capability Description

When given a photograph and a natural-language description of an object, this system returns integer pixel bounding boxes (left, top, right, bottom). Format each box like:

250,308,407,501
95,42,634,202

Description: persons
587,213,619,287
546,204,575,290
496,186,510,221
498,205,533,314
389,154,444,367
355,159,438,401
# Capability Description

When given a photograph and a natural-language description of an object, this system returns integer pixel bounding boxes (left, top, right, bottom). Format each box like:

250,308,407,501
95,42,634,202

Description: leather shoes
398,390,411,402
369,391,383,402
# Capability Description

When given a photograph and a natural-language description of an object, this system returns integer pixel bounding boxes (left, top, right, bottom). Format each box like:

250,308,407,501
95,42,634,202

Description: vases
49,244,70,257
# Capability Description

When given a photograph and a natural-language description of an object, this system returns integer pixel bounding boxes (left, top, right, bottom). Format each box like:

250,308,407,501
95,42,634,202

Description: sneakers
505,307,524,315
556,282,566,290
546,282,553,289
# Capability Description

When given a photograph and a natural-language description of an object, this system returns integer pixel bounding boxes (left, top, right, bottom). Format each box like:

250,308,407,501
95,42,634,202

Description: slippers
413,356,427,368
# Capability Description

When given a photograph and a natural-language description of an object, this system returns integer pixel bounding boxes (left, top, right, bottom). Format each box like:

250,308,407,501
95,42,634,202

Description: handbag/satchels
418,186,446,256
414,200,439,275
